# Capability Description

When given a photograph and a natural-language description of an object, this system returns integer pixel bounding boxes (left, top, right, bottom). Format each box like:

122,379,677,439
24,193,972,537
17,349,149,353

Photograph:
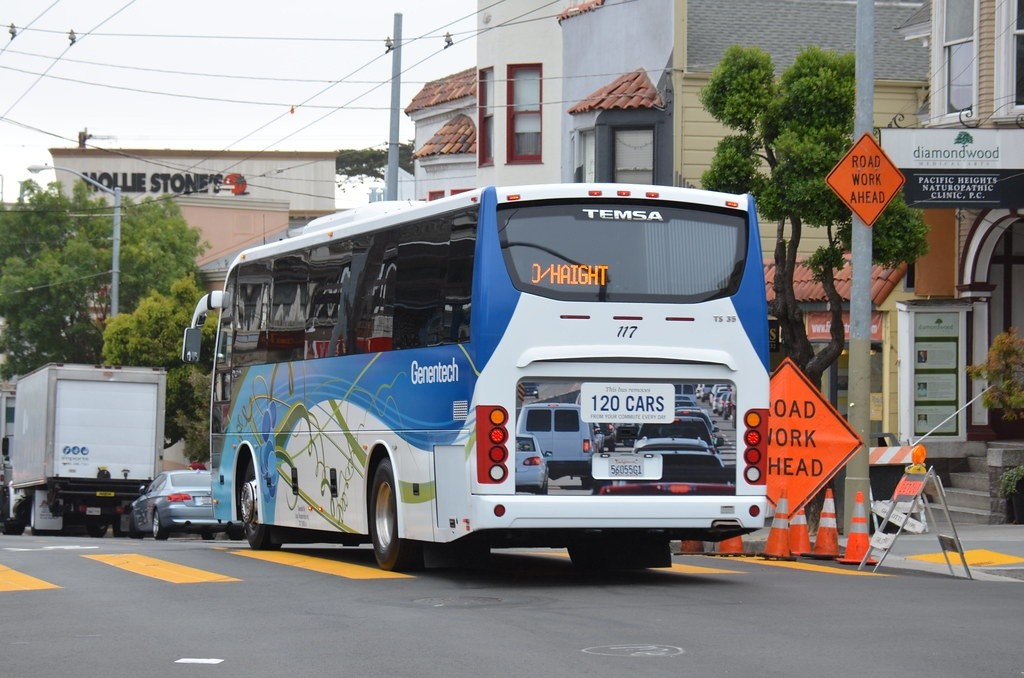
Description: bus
181,182,771,583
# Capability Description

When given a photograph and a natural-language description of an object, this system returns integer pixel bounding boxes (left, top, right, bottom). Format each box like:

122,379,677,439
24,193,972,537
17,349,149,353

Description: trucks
0,361,168,538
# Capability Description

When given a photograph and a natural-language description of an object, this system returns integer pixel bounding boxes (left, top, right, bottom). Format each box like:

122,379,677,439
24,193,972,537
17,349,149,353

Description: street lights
27,162,122,320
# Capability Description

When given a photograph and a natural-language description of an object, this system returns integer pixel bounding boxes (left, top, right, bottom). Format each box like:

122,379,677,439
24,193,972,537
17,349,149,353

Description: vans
517,402,604,491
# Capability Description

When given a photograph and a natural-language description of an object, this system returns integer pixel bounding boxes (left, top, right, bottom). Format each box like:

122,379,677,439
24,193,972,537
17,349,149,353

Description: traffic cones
758,487,797,562
711,536,755,557
799,488,844,560
786,505,811,556
835,491,878,566
672,540,707,555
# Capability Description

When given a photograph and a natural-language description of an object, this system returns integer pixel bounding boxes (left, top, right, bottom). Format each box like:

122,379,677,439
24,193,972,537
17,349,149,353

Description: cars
129,468,244,541
574,384,738,496
515,434,553,495
